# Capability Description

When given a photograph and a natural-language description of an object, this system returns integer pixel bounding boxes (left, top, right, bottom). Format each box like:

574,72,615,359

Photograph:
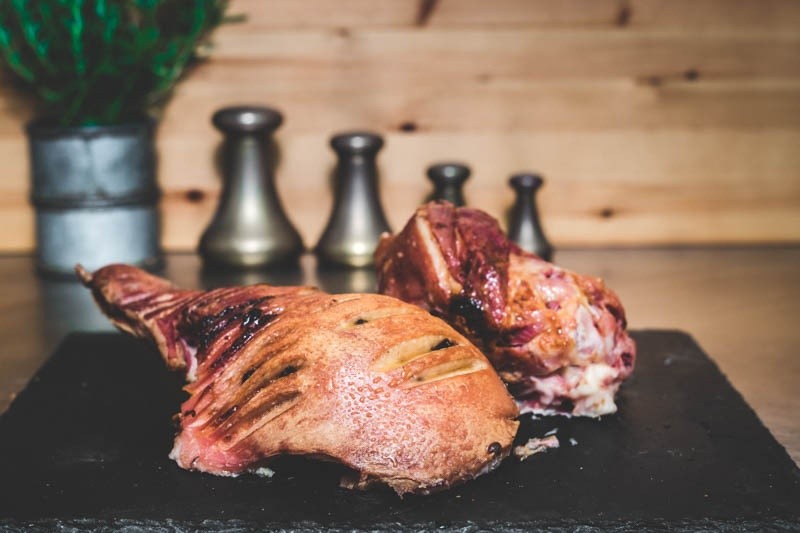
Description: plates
0,327,800,533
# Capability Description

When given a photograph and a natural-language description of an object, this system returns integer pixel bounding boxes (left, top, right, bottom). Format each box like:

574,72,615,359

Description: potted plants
0,0,245,288
